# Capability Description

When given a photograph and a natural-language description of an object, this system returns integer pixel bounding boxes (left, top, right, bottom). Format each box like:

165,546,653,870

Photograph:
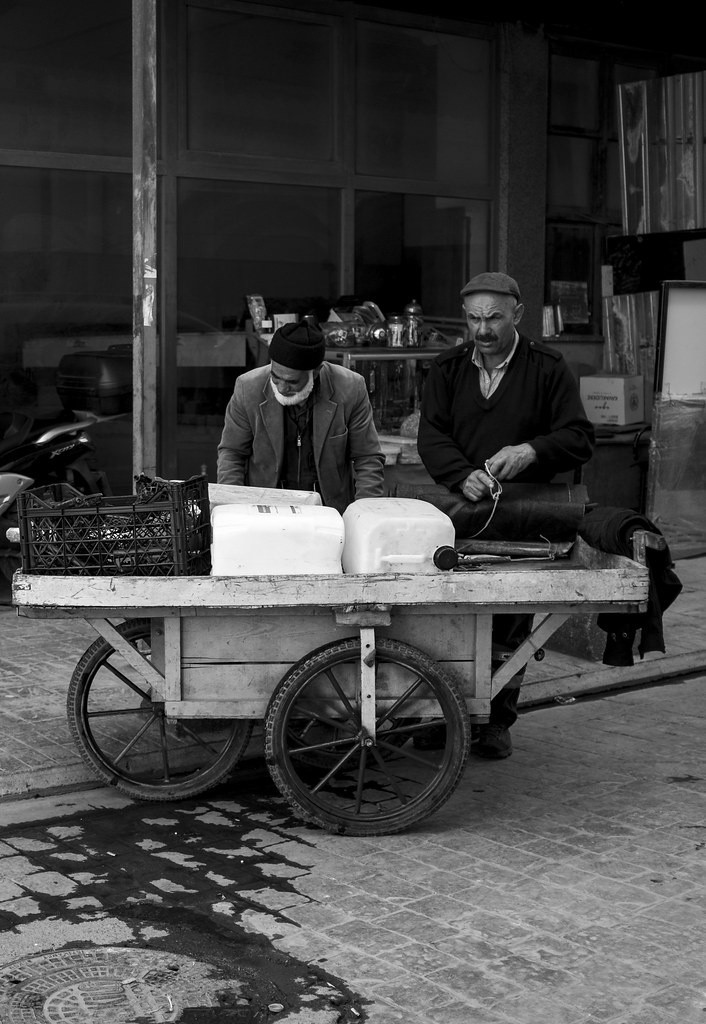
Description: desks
319,339,459,433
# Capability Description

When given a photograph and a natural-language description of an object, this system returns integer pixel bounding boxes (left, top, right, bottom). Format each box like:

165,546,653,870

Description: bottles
387,305,425,347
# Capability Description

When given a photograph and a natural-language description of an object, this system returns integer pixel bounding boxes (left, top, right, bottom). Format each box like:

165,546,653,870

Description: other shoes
413,718,480,751
480,724,513,760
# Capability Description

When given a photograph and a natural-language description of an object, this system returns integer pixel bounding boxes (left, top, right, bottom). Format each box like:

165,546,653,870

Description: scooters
0,343,132,584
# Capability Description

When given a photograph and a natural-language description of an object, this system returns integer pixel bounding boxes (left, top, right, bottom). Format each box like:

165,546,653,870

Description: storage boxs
174,328,248,392
16,470,207,577
579,373,644,426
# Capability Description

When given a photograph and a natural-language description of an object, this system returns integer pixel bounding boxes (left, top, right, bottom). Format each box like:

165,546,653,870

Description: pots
50,343,135,417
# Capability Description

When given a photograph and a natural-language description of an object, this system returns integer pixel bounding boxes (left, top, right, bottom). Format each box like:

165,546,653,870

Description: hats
460,273,521,306
269,320,325,371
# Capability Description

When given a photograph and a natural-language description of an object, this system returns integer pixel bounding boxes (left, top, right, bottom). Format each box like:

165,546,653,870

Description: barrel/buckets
160,480,344,576
341,496,458,574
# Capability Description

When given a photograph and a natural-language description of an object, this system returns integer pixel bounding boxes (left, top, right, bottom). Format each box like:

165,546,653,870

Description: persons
412,272,595,759
217,320,386,517
